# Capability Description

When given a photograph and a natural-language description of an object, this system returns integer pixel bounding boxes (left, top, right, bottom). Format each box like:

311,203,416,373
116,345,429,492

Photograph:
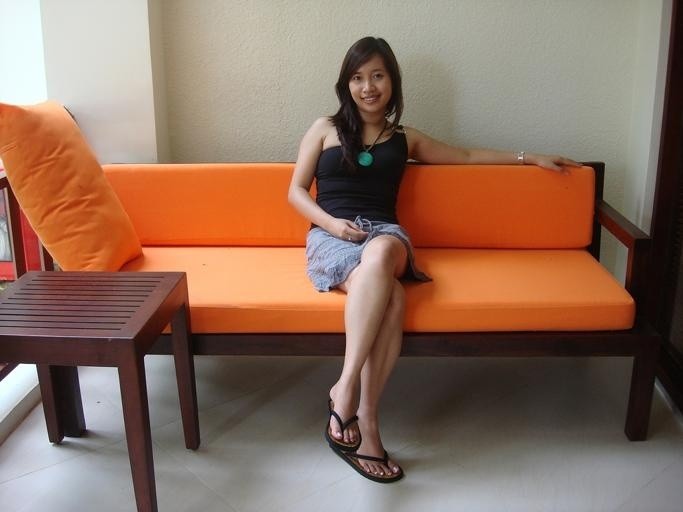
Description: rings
348,237,351,240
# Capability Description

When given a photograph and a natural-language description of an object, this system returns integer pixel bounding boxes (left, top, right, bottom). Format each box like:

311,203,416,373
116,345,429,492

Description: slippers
325,398,403,483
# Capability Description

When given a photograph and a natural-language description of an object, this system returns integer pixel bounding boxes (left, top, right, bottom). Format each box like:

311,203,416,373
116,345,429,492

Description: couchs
0,158,660,440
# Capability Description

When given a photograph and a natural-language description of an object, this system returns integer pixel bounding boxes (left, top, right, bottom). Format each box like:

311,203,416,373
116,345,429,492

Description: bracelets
517,151,525,164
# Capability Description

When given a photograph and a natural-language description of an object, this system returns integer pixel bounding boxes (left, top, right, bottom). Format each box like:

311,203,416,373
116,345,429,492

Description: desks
0,271,200,510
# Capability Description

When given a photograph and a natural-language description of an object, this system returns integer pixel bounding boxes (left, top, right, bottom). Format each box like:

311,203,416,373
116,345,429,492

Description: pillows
0,101,143,271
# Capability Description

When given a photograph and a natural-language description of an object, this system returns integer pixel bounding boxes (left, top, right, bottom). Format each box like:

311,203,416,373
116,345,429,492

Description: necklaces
355,116,388,167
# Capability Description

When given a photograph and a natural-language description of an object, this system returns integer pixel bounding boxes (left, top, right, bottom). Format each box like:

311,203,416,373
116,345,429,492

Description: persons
286,35,586,485
